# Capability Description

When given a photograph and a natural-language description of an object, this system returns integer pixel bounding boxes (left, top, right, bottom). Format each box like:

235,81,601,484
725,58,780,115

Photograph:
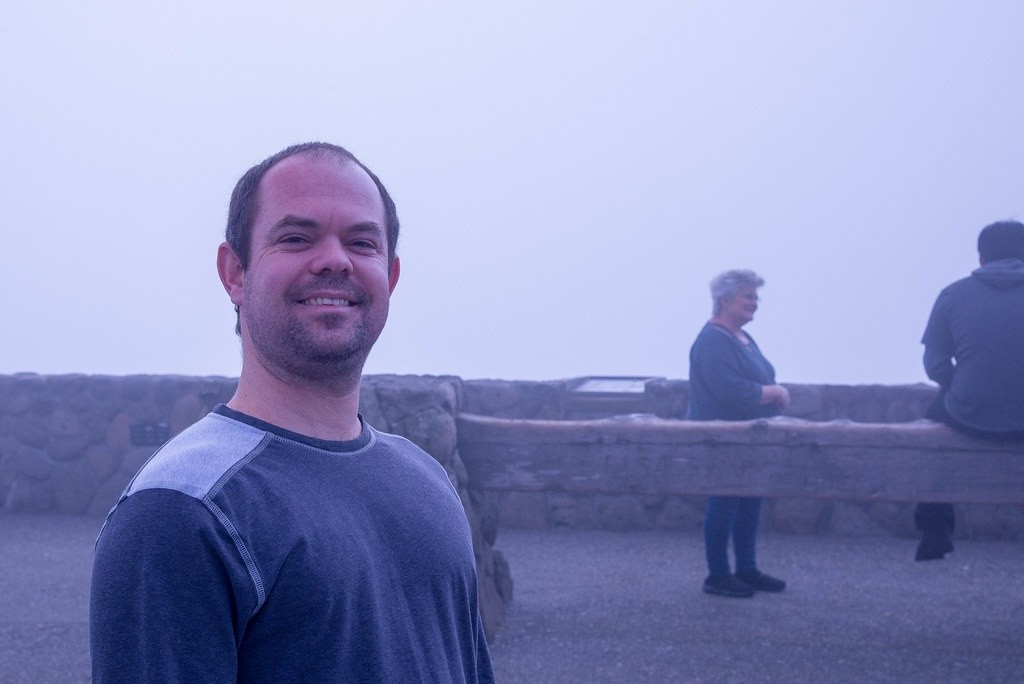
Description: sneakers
732,570,787,594
914,529,955,562
701,575,756,599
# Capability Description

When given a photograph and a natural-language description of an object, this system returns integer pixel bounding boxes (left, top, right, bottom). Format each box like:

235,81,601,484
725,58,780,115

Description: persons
913,219,1024,563
682,266,794,599
84,137,493,682
